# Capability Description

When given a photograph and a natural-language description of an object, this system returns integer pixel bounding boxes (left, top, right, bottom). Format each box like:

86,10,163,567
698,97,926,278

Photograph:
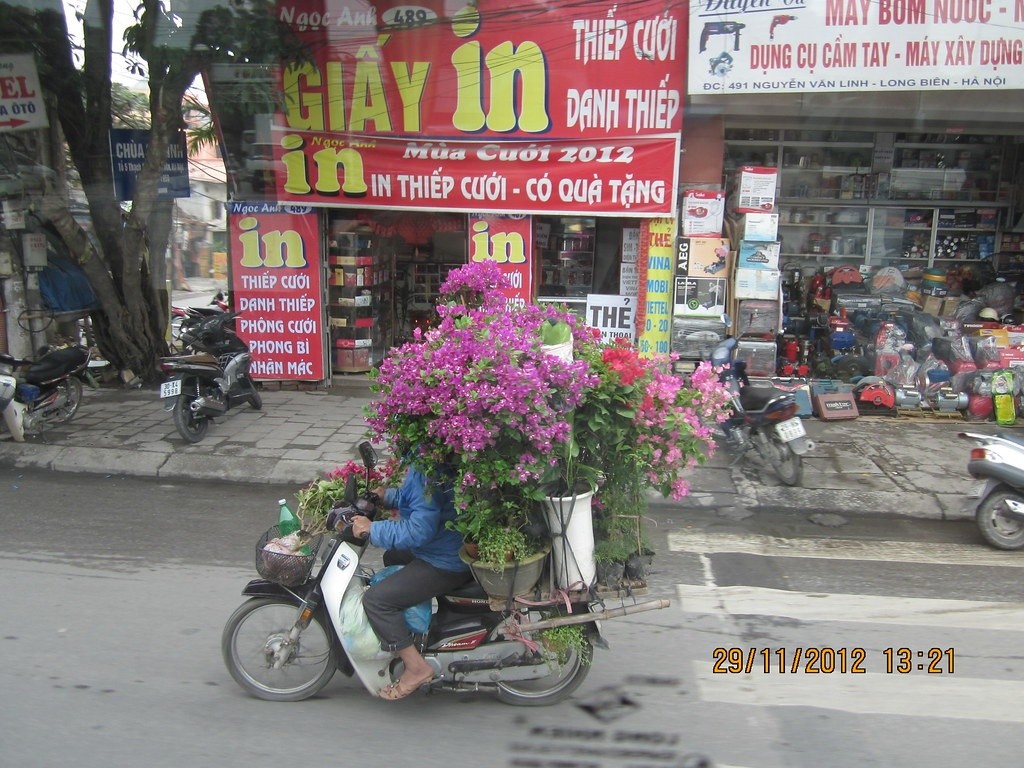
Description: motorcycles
159,310,262,443
699,311,815,487
0,344,100,445
957,431,1024,551
170,287,231,341
219,441,611,707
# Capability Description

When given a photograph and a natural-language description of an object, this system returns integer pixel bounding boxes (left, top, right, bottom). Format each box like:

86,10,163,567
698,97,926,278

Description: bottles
279,499,311,556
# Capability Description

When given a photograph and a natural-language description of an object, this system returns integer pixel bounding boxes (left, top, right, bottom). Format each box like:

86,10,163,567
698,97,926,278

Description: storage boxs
921,295,960,317
962,322,1024,368
671,165,781,373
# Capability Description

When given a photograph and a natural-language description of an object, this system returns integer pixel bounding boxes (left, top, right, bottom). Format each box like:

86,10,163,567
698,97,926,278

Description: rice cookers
789,208,831,223
809,233,855,254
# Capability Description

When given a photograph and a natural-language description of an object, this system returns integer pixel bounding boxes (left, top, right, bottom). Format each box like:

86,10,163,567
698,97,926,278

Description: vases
625,549,658,580
534,480,599,592
462,532,516,562
596,559,627,586
524,519,545,536
458,540,553,599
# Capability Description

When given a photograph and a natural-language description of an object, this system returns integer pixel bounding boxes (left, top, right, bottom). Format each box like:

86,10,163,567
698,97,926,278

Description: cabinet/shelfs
538,218,597,296
328,230,464,375
726,133,1024,275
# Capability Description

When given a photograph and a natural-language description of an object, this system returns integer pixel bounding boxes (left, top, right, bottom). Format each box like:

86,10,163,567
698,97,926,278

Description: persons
350,413,472,700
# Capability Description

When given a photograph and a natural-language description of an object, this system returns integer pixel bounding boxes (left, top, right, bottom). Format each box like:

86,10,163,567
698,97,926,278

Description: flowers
262,459,397,578
365,259,743,573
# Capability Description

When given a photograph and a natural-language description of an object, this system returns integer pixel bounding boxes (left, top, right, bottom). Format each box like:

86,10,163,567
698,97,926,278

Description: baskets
255,524,323,587
0,381,15,410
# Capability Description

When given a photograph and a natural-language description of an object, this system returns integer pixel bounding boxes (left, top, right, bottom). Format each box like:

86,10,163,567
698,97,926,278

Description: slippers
377,669,435,701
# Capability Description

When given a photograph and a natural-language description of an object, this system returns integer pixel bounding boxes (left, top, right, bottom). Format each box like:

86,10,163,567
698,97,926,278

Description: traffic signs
0,52,50,133
